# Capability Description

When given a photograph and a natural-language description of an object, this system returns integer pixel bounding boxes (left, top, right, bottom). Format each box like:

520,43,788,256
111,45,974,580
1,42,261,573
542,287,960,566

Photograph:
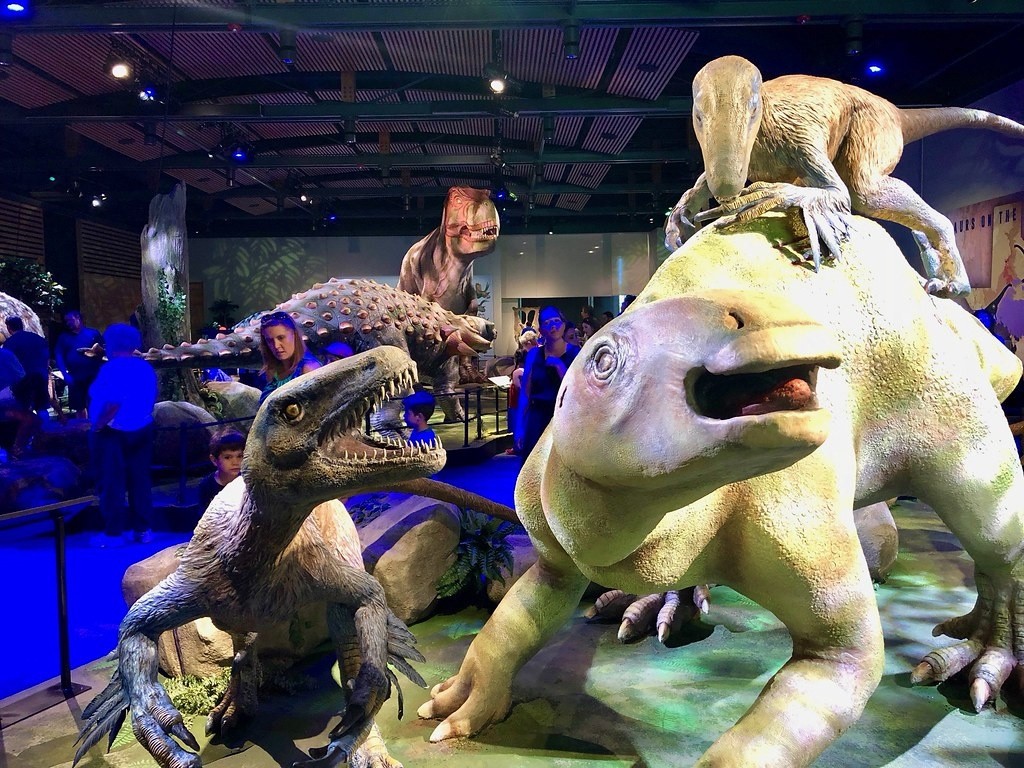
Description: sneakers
138,528,157,543
89,531,126,548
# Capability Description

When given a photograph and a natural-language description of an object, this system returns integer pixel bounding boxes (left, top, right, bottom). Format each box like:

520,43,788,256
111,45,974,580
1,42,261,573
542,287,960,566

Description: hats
317,340,354,358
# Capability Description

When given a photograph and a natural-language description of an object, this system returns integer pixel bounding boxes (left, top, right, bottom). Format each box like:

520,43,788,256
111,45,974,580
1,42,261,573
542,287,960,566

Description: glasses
261,311,295,329
583,318,593,325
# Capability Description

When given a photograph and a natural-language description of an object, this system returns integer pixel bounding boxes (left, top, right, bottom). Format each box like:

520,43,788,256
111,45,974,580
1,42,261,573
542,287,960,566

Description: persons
192,310,327,644
0,309,159,552
505,295,638,463
399,391,441,482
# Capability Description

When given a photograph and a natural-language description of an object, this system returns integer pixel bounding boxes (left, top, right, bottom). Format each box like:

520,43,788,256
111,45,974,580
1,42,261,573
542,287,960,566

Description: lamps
499,106,519,121
208,134,230,159
483,63,507,94
102,42,129,79
89,185,107,209
490,152,506,169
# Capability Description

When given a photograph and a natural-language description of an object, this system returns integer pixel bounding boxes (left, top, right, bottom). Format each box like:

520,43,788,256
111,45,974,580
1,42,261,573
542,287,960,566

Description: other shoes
506,446,521,455
